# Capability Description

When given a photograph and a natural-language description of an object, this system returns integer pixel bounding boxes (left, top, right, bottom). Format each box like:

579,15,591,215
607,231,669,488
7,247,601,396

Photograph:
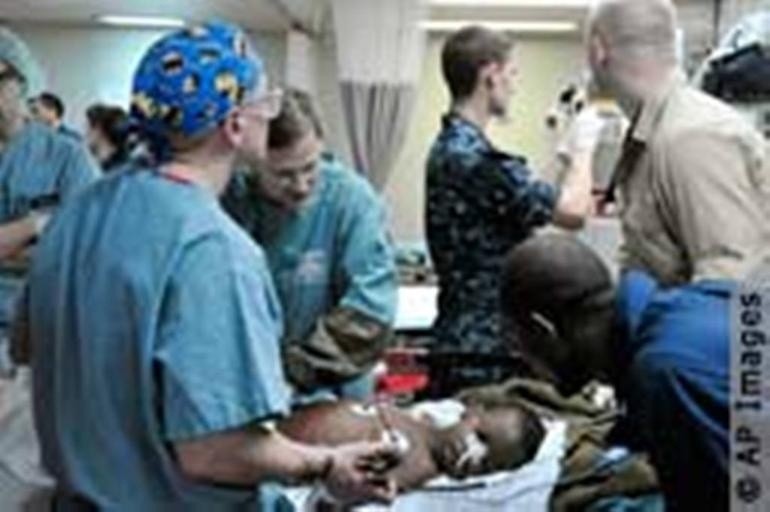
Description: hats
130,20,265,162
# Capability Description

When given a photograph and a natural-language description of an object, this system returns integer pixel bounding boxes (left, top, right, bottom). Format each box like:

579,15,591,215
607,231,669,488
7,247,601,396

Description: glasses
241,87,285,119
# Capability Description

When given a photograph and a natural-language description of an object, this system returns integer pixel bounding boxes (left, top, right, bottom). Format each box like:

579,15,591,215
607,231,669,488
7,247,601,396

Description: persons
0,0,770,511
